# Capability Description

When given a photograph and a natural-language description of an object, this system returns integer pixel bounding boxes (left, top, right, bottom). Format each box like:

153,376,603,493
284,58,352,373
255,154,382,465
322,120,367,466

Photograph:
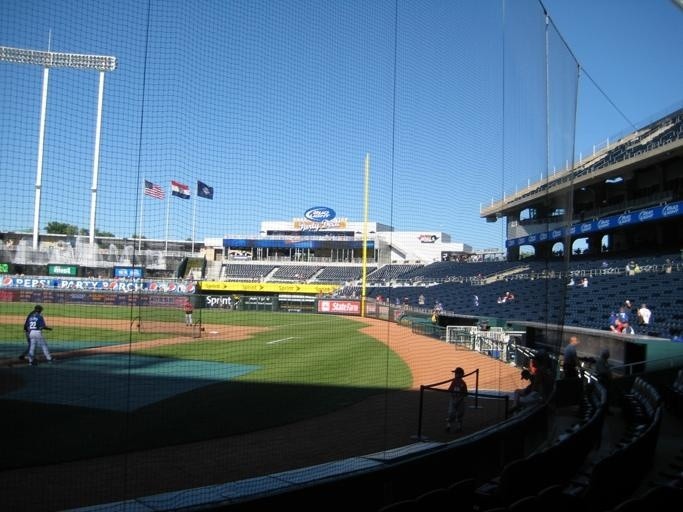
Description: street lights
0,46,118,252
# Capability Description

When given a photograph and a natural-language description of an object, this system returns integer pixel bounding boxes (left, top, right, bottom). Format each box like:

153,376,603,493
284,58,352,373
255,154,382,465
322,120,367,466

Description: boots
456,422,462,431
447,421,451,429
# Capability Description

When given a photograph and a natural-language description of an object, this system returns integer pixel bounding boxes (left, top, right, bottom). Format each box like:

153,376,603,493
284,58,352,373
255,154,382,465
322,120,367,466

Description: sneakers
18,353,56,366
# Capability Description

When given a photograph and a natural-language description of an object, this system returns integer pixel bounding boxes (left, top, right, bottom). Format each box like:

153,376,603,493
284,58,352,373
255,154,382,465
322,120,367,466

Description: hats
452,368,462,373
521,370,529,379
529,355,543,363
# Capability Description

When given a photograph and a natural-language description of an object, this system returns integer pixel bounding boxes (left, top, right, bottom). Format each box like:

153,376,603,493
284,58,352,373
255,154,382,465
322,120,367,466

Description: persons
376,291,514,314
506,335,611,412
18,309,34,359
446,367,467,437
567,257,672,337
180,299,194,326
23,305,56,363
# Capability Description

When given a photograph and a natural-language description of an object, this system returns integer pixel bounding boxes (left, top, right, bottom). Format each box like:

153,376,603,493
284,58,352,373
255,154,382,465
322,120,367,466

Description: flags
196,180,213,200
171,180,189,200
143,180,164,201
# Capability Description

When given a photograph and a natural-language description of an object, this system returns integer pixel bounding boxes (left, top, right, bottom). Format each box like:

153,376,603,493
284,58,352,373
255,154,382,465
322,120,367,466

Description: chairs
384,369,683,512
